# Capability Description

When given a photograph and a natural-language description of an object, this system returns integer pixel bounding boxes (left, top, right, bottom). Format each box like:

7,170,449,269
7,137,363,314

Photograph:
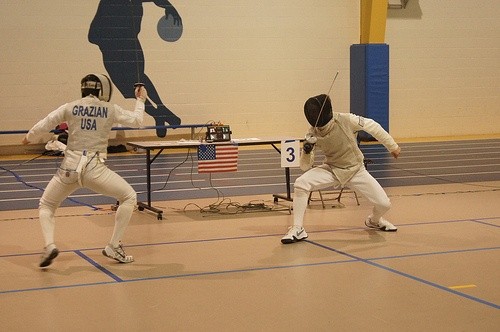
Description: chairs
308,129,365,208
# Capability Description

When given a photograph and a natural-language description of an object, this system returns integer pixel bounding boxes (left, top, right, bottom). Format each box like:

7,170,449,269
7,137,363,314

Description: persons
22,73,147,267
281,94,402,243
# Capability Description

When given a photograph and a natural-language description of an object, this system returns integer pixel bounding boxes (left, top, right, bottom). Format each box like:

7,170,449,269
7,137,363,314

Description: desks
129,137,308,220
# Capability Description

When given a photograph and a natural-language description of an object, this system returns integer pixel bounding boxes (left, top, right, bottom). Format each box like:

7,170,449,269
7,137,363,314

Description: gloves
303,143,314,153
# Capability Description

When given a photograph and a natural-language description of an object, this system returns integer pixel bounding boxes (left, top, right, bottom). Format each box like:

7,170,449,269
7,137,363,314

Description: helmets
80,74,112,102
304,94,333,127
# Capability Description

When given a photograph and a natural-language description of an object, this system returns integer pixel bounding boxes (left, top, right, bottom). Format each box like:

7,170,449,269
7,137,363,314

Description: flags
197,144,238,173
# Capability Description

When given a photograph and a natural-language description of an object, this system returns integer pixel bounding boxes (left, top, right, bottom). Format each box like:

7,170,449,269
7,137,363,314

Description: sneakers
365,215,397,231
102,241,134,262
40,244,58,267
281,226,308,243
